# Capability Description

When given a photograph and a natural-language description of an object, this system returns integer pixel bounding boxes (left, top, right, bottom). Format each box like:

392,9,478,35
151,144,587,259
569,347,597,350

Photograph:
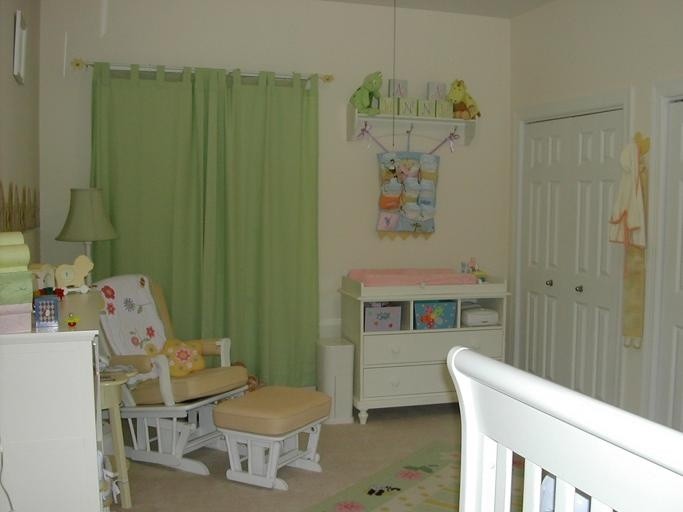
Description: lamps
53,186,120,286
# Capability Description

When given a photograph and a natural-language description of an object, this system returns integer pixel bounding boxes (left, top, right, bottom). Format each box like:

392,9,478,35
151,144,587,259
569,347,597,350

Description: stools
98,369,136,508
211,384,332,490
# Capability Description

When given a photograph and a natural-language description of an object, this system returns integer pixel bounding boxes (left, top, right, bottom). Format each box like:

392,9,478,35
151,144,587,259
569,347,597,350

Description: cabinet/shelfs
0,289,105,511
337,269,513,424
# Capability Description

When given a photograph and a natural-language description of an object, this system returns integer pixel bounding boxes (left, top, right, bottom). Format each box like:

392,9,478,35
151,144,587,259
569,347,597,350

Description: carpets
304,440,523,511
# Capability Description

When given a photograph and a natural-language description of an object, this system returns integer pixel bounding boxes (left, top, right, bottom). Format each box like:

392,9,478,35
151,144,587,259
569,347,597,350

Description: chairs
94,277,249,478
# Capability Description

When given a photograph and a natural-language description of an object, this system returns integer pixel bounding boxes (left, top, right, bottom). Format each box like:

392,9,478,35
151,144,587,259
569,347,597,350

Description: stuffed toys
445,78,482,121
350,70,384,115
